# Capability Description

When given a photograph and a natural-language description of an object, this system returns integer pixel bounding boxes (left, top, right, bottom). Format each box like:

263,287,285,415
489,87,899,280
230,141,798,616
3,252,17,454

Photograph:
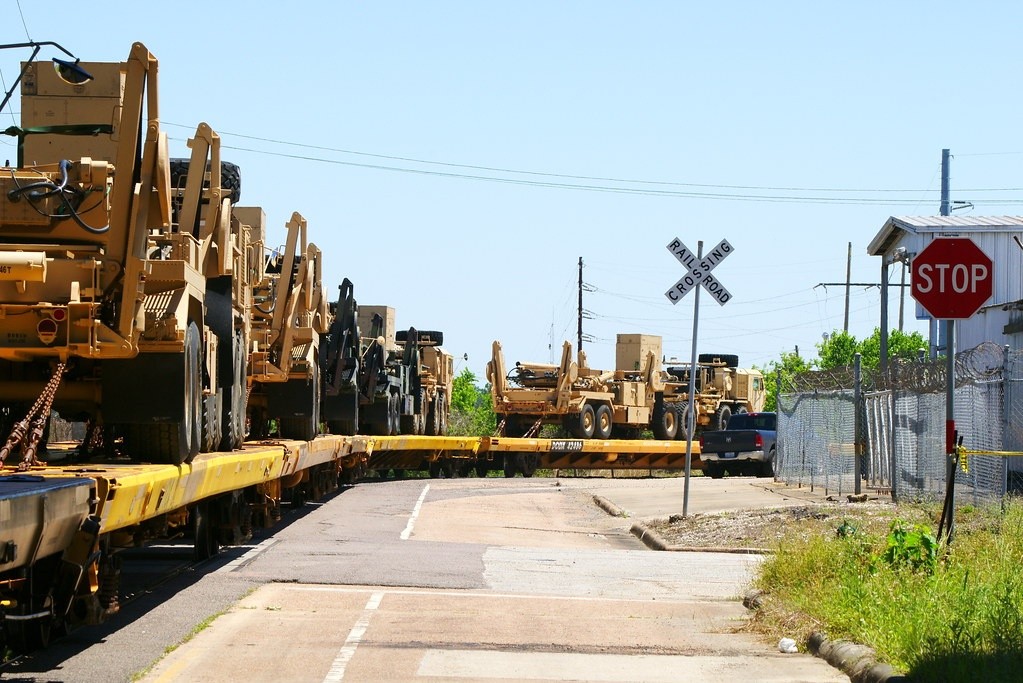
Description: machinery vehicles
1,41,454,464
486,333,766,439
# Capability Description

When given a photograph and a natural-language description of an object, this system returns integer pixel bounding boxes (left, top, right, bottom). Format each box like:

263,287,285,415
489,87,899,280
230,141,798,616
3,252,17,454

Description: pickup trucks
699,411,826,477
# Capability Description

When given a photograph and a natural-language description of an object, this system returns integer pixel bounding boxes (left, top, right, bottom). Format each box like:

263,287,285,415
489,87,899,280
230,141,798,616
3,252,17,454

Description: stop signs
911,235,995,321
